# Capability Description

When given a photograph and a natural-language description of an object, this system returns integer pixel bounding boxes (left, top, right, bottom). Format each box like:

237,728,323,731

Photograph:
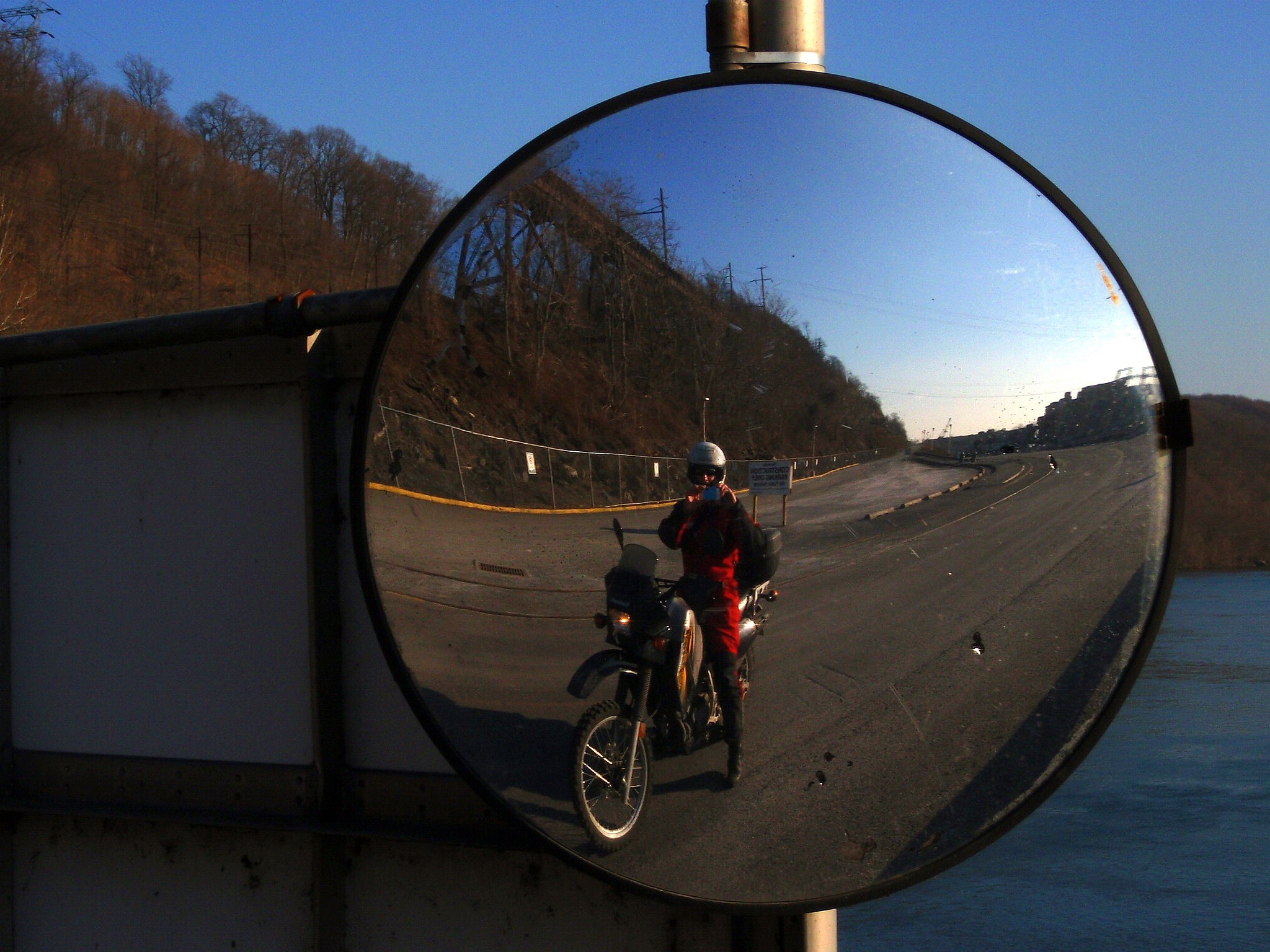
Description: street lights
811,425,819,475
701,397,710,442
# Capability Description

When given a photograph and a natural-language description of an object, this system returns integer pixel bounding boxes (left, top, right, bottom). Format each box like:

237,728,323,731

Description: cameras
703,487,720,501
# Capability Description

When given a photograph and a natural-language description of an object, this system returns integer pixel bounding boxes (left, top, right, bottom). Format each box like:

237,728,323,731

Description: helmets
687,442,726,487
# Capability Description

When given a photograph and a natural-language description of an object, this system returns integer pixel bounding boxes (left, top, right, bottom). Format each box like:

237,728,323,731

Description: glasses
694,467,716,476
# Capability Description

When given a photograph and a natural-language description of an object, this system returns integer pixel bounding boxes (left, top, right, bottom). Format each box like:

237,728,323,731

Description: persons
656,441,759,788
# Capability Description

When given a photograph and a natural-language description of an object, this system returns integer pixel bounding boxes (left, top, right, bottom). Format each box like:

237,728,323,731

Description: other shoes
727,757,741,784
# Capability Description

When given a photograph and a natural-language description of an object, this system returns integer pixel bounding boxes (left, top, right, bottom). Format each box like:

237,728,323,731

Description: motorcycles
561,515,785,855
